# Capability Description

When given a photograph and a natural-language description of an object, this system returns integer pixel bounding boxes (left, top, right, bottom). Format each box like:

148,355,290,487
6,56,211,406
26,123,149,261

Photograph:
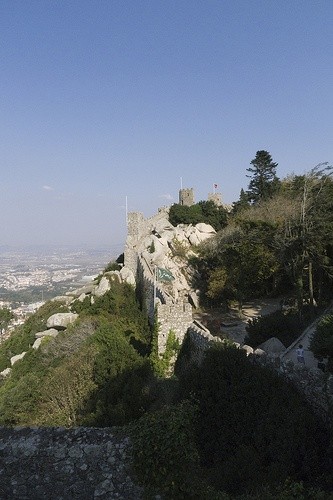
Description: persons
295,344,305,363
